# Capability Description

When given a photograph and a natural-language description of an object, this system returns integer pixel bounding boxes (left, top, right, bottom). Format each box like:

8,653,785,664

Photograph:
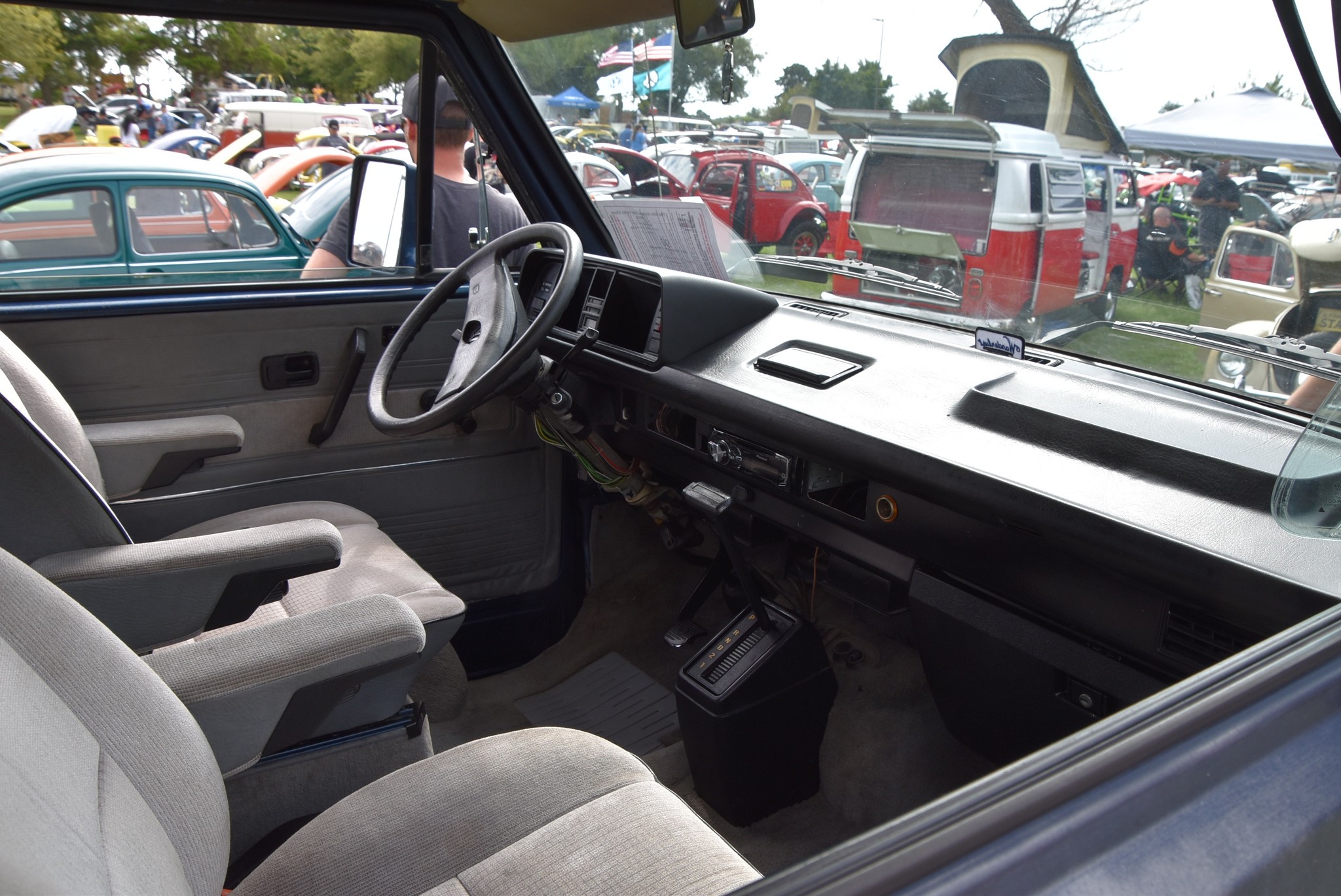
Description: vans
826,106,1141,347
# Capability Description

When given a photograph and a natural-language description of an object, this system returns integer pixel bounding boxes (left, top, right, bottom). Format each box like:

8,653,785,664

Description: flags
595,66,633,95
633,30,673,62
633,60,671,96
597,40,633,68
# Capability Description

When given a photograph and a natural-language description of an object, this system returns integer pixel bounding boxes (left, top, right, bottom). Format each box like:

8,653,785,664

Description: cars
588,141,832,260
1085,152,1341,408
1,146,434,294
0,0,869,243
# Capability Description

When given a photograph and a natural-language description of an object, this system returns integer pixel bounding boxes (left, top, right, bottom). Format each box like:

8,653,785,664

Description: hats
329,119,339,130
390,73,474,131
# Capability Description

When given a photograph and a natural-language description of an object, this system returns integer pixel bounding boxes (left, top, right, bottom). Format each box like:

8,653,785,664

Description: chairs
1133,233,1202,306
0,536,764,896
129,206,155,255
89,201,117,254
0,328,468,882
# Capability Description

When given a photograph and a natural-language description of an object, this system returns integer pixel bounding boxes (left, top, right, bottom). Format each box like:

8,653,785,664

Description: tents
545,86,601,125
1123,86,1341,223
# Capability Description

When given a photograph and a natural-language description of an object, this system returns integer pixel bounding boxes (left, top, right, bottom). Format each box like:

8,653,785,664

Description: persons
1136,206,1208,297
298,70,543,285
464,128,506,195
618,122,633,149
1189,155,1241,280
572,112,580,123
629,123,646,153
1282,337,1341,414
316,119,354,181
16,75,400,152
557,114,567,126
1227,218,1291,285
